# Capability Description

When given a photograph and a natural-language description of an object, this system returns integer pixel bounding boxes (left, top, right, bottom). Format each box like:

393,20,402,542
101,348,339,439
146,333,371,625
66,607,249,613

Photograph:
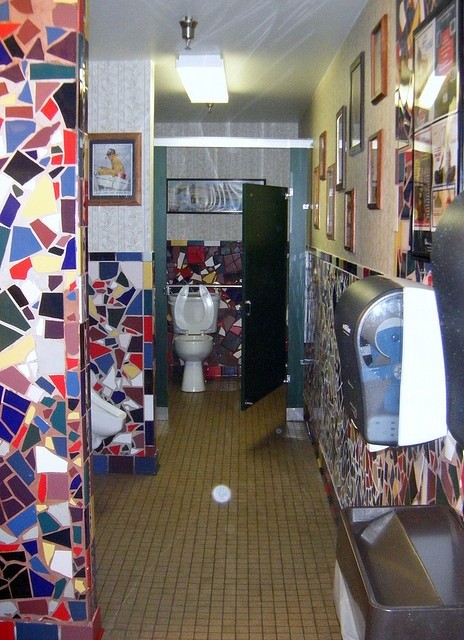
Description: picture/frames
167,179,267,215
312,167,320,230
344,189,357,252
335,105,348,191
325,163,337,240
86,132,142,205
364,129,383,208
347,51,364,153
368,13,391,106
412,0,463,263
318,131,327,182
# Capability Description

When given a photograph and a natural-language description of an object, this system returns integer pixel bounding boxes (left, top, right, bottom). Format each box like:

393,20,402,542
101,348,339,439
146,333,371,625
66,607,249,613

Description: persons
98,149,125,179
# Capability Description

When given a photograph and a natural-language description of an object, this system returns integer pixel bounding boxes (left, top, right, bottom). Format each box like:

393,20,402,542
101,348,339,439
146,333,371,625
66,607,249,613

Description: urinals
91,386,128,452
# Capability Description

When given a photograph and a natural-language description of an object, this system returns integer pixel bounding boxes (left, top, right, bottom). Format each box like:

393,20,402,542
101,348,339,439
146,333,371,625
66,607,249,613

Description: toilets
169,284,222,393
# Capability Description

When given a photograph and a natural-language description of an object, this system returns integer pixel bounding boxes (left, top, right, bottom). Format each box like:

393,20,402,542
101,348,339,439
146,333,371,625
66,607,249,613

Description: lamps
174,55,228,104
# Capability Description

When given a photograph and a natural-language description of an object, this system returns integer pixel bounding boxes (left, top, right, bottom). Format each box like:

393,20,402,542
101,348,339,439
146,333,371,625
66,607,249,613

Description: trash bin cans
332,503,464,640
